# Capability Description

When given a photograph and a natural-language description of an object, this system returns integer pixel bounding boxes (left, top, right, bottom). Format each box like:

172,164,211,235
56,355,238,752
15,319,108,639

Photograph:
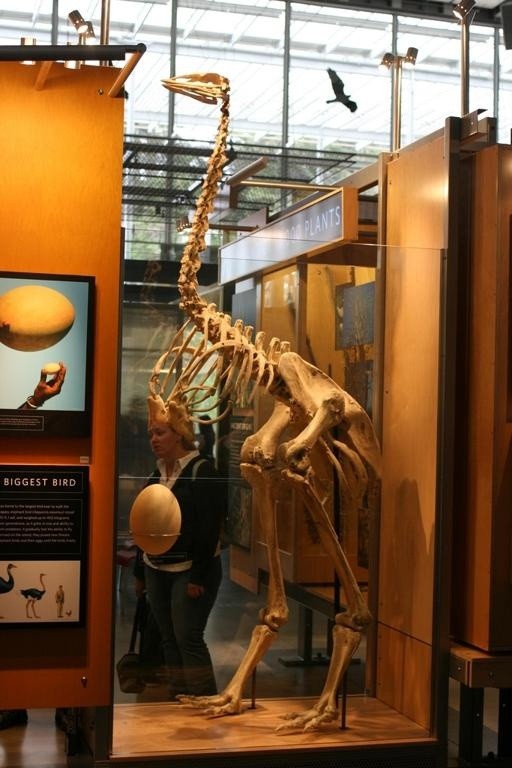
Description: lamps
69,8,95,70
452,0,478,118
380,48,417,154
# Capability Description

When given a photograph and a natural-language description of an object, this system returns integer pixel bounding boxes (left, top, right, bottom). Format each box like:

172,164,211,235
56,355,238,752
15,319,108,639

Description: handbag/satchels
118,652,146,693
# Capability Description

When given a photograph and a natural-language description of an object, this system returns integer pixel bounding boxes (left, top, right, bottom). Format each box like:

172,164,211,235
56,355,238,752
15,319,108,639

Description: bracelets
27,396,39,408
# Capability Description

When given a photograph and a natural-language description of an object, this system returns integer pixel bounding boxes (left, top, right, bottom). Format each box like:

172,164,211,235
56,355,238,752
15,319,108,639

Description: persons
137,418,223,700
56,585,64,617
17,362,66,410
137,569,164,688
199,415,216,471
